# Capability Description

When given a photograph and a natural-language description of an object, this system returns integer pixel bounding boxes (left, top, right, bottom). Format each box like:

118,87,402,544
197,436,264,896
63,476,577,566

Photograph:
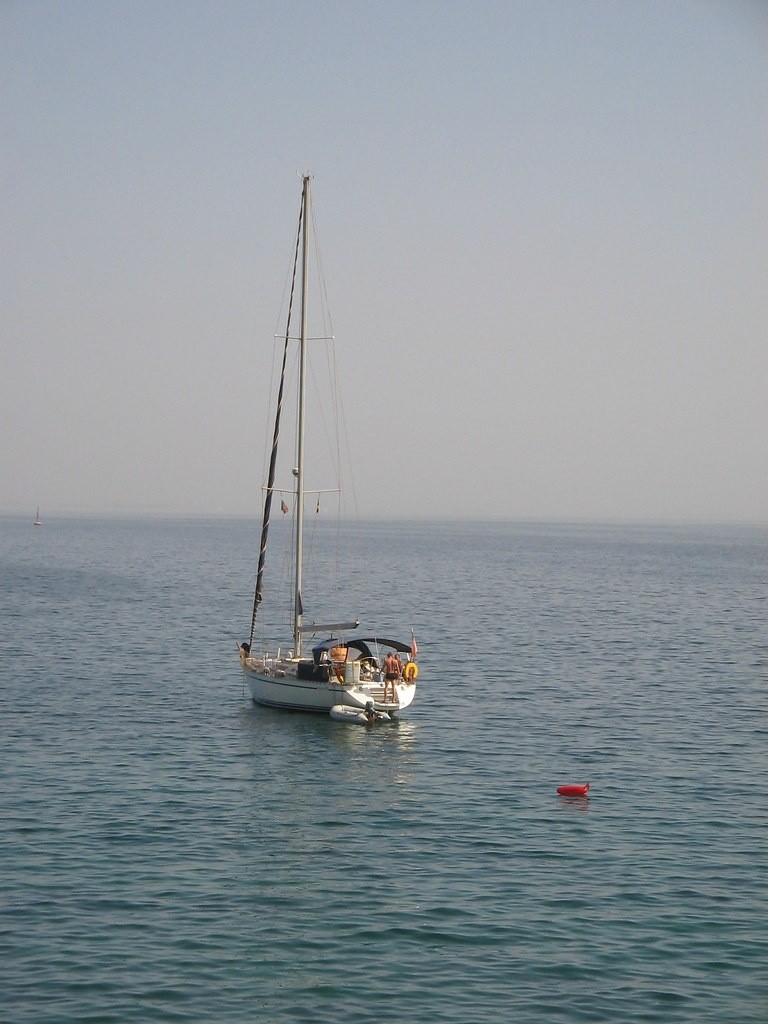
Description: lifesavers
404,663,418,678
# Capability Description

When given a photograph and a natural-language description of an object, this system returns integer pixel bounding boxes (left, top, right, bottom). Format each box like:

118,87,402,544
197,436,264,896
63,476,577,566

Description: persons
379,652,404,703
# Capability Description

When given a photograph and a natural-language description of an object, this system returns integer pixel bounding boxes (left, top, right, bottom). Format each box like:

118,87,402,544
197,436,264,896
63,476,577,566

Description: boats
329,704,392,724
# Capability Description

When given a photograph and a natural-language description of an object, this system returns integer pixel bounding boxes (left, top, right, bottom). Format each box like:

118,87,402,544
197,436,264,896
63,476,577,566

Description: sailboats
34,506,41,525
239,171,418,715
